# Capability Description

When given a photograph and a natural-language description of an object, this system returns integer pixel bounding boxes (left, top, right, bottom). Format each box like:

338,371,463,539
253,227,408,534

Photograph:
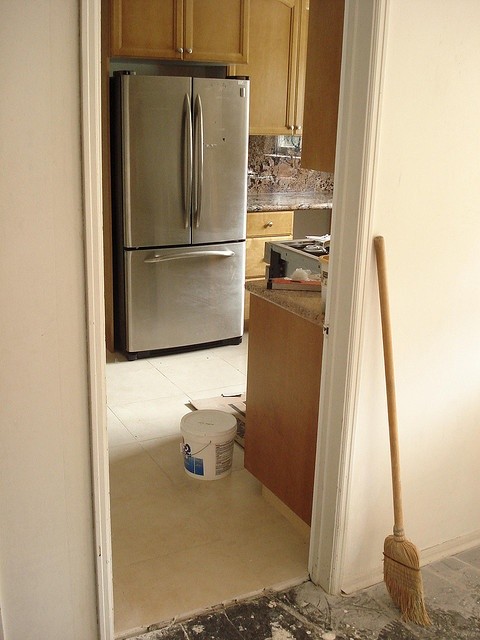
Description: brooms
369,236,433,626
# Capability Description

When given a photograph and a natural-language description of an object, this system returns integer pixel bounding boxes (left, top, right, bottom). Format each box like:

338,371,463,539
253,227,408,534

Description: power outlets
278,134,301,148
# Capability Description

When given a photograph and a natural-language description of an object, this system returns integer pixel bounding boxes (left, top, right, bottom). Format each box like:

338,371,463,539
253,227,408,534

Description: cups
319,255,330,314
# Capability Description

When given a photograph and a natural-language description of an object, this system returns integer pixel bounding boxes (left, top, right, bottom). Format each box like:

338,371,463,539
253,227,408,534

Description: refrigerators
112,71,249,361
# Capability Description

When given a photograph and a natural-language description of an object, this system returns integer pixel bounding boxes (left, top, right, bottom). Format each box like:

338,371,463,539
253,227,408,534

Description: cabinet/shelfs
227,0,309,137
245,211,294,319
108,0,249,65
299,0,345,171
243,291,324,540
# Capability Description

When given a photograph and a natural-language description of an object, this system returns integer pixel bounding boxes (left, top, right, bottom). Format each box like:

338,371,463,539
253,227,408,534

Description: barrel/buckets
178,408,241,481
